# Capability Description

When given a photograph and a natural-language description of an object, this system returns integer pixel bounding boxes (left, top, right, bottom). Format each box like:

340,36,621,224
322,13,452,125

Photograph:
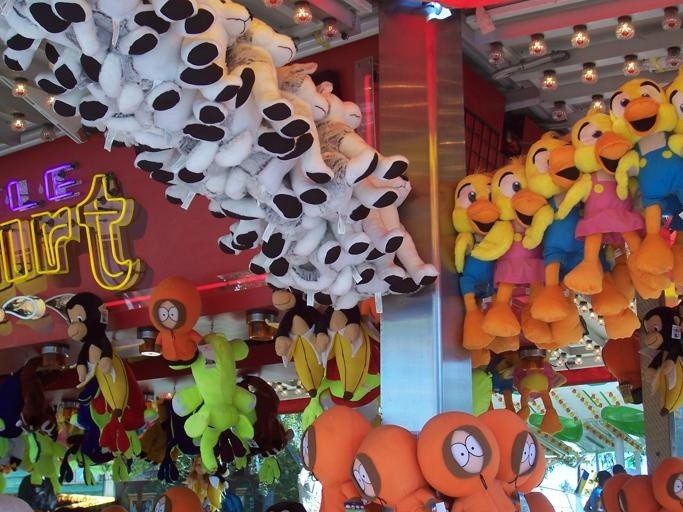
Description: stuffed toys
0,0,683,512
